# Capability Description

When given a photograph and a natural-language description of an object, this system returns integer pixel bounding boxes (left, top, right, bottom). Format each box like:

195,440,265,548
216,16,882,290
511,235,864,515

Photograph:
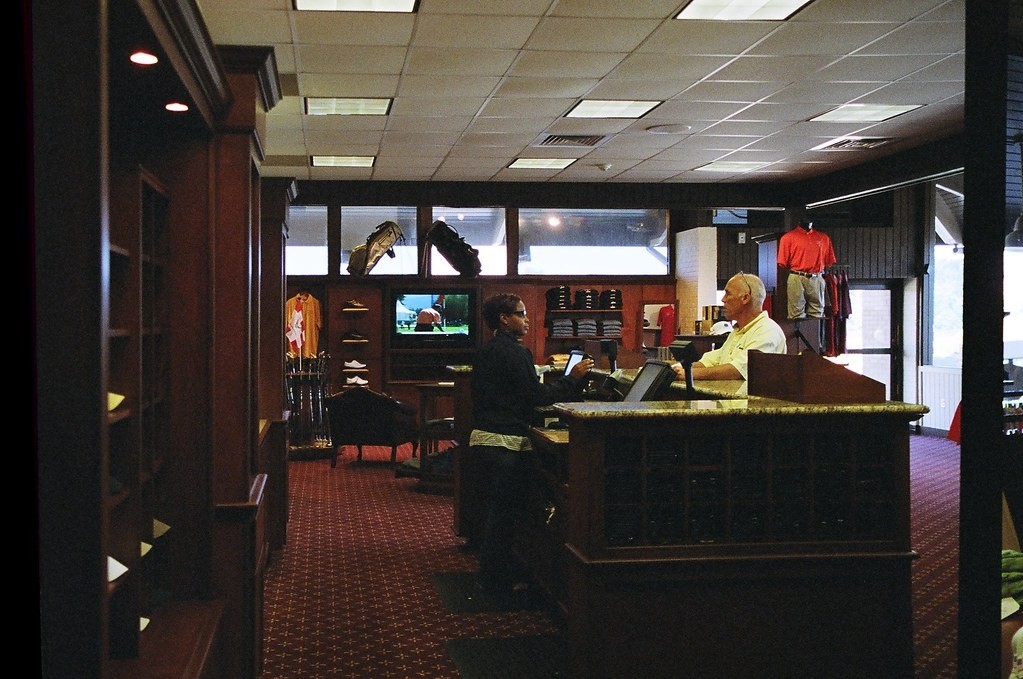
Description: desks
420,383,455,484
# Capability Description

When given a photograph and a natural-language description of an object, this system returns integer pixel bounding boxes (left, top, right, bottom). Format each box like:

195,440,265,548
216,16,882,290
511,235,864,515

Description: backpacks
347,221,406,280
426,220,482,276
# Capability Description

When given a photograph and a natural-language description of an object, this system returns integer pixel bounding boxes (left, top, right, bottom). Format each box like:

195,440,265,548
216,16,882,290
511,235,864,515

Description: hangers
823,263,849,277
300,288,309,299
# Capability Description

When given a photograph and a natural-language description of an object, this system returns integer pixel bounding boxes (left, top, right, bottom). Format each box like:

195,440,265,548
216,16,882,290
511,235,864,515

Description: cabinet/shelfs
340,306,370,389
33,0,299,679
549,308,624,342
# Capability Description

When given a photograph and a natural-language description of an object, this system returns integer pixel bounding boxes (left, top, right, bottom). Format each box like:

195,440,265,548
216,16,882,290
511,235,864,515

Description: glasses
514,311,526,316
737,271,752,297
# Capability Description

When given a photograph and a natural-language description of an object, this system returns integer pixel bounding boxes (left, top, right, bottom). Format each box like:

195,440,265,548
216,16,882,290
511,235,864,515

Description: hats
433,304,443,311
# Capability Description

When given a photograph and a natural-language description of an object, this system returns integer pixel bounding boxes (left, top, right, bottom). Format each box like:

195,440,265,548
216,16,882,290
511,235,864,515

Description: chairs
324,384,417,468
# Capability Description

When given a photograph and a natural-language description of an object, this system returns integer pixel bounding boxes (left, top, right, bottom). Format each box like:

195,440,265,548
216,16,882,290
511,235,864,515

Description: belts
790,270,823,277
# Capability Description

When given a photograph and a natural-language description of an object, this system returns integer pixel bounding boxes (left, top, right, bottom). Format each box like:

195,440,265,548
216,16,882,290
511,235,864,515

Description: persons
672,271,787,380
414,304,445,332
657,304,674,346
459,293,595,600
779,221,836,317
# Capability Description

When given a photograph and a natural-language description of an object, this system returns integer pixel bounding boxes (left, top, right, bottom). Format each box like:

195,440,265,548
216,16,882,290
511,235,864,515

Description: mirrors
638,300,680,352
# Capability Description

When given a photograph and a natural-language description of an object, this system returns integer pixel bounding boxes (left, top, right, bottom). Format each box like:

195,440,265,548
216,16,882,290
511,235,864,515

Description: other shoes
344,299,366,308
345,328,368,339
346,376,368,385
344,360,367,369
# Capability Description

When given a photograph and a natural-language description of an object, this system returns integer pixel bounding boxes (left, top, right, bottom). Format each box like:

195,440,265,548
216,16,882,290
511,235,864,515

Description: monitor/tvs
622,360,676,402
563,349,595,392
391,289,477,349
